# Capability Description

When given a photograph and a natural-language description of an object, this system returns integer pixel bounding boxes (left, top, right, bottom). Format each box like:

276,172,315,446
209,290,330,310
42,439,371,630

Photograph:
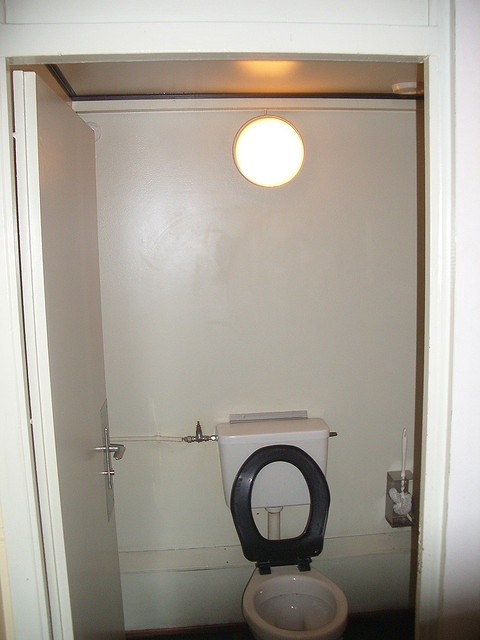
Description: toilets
215,418,349,640
